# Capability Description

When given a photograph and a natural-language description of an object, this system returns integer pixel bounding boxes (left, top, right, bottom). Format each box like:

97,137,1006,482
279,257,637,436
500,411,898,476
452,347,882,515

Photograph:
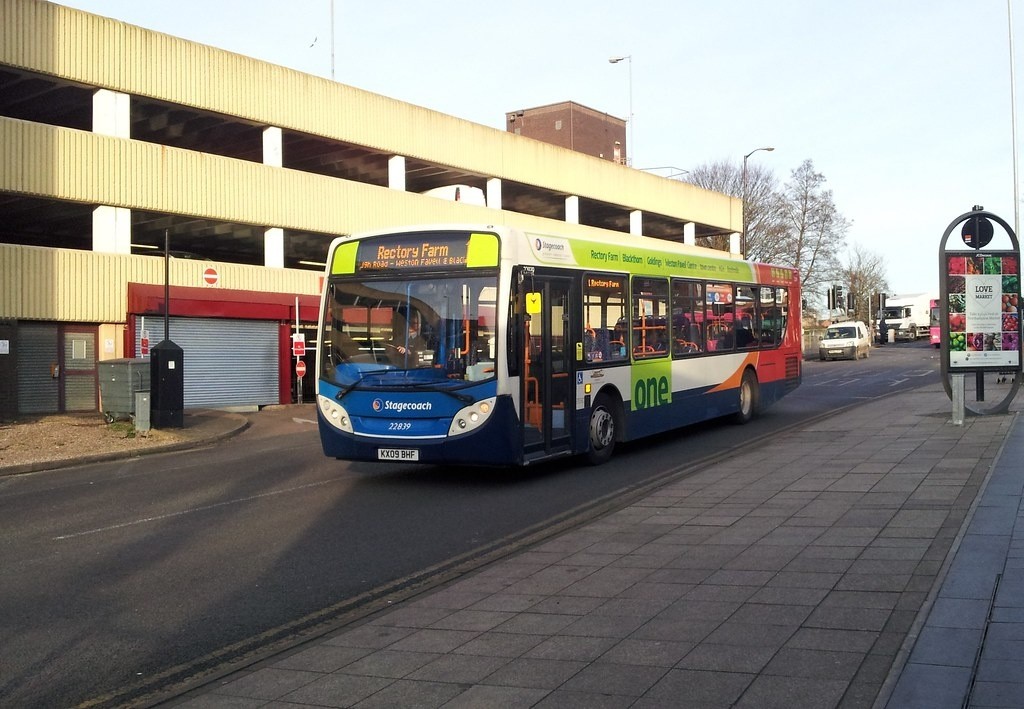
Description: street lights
610,54,633,168
744,148,775,259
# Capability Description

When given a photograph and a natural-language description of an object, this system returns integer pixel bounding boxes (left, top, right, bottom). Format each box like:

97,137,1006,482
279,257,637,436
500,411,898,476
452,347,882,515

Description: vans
819,320,869,361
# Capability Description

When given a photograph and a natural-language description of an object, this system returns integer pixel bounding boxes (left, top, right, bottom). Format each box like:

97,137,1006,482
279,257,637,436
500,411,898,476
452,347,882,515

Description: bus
314,222,803,476
926,299,940,349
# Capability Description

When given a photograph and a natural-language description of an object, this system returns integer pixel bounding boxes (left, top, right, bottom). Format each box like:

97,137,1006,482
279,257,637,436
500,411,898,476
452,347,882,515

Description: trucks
875,292,934,343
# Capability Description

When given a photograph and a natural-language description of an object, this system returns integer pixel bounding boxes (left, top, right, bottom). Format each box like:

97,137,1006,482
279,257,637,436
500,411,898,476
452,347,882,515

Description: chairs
585,310,753,362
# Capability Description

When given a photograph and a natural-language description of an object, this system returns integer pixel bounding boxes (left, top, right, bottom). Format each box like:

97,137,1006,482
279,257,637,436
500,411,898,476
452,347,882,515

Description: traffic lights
802,300,807,309
833,285,843,308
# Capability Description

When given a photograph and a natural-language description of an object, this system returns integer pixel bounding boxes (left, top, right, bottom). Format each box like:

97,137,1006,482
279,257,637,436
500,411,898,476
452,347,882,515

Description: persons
834,332,840,338
880,317,887,345
585,330,600,351
606,323,625,357
380,312,425,370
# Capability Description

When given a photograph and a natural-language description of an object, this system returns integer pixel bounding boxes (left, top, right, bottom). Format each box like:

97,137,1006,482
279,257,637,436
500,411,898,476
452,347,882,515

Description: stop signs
203,269,219,284
296,362,306,377
